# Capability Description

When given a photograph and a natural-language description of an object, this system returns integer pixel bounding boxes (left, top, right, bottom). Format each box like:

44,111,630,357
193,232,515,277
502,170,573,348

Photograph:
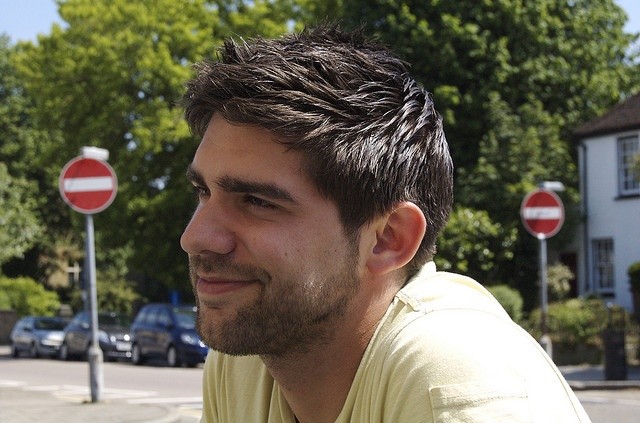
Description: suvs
127,301,210,367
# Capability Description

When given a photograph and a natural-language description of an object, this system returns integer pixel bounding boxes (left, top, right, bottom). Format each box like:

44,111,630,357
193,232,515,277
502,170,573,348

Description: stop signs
520,186,566,239
58,154,119,214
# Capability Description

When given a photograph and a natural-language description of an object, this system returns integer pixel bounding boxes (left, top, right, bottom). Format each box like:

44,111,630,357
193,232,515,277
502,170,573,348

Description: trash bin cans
602,326,626,381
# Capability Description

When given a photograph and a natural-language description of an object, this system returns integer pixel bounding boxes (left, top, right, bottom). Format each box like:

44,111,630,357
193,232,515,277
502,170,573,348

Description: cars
59,309,132,360
10,315,66,358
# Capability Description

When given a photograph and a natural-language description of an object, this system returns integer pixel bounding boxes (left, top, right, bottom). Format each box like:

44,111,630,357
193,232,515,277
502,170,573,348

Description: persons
180,18,593,423
